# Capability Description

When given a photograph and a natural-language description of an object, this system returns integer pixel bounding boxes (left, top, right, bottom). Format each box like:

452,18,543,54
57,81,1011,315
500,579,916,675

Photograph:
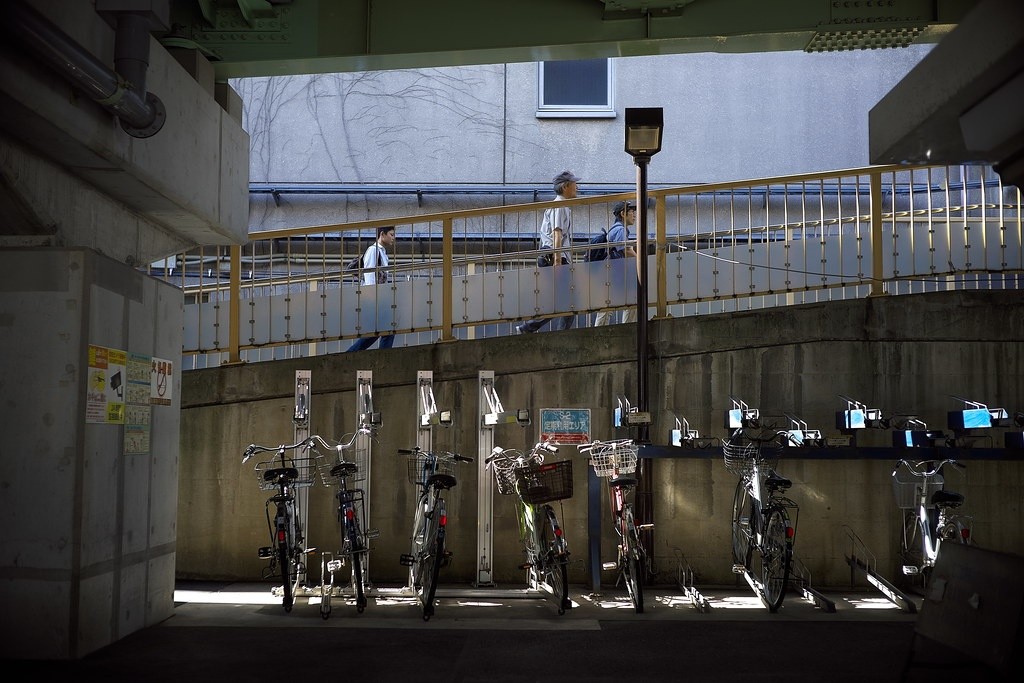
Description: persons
515,170,582,334
594,201,636,327
345,226,397,351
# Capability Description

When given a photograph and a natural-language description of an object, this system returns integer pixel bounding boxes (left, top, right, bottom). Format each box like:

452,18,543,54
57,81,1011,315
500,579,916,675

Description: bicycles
892,456,973,595
240,436,318,612
722,413,803,613
576,439,657,614
301,414,382,615
484,435,575,611
395,447,475,618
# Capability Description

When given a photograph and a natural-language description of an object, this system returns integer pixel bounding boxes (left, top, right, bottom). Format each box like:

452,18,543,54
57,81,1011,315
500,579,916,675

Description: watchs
555,258,562,264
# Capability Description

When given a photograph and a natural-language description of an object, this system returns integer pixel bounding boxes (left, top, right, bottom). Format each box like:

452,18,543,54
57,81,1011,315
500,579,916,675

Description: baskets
408,458,456,486
492,454,544,495
316,448,366,487
722,435,783,474
590,438,637,477
892,472,944,510
514,460,574,504
254,457,317,487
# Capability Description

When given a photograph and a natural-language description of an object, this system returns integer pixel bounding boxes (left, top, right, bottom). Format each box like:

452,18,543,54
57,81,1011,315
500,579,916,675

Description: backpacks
584,225,629,262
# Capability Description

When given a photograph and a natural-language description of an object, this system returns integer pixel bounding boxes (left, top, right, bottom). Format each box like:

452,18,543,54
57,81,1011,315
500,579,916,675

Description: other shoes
515,325,523,334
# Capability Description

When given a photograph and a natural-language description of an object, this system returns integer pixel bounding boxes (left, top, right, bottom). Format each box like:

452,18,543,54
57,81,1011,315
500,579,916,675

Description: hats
552,171,582,185
613,200,636,216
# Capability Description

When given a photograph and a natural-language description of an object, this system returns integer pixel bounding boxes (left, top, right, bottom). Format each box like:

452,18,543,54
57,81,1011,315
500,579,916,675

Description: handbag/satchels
346,245,387,284
537,246,554,267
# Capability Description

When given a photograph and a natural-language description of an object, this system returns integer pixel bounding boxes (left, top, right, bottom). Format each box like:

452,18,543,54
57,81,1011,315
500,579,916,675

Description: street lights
622,106,666,587
